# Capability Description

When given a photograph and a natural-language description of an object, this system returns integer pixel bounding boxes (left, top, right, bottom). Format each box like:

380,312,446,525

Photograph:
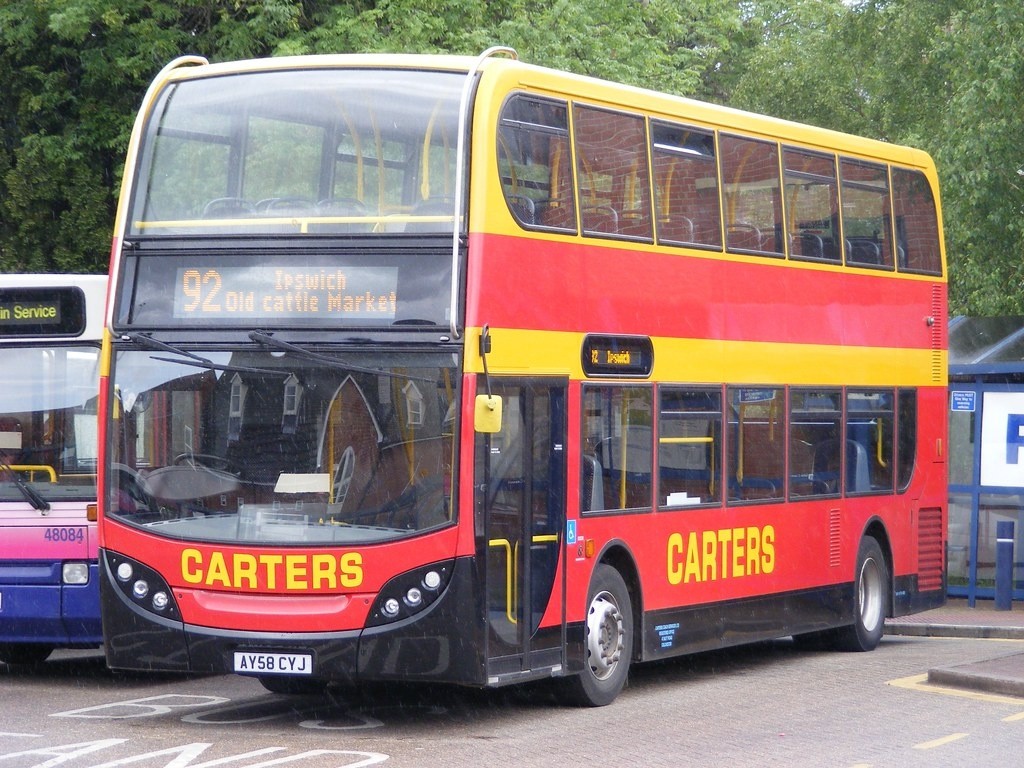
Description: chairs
199,194,906,267
520,419,871,589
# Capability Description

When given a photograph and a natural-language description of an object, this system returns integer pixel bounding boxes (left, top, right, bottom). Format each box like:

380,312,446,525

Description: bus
90,46,950,706
0,273,224,675
